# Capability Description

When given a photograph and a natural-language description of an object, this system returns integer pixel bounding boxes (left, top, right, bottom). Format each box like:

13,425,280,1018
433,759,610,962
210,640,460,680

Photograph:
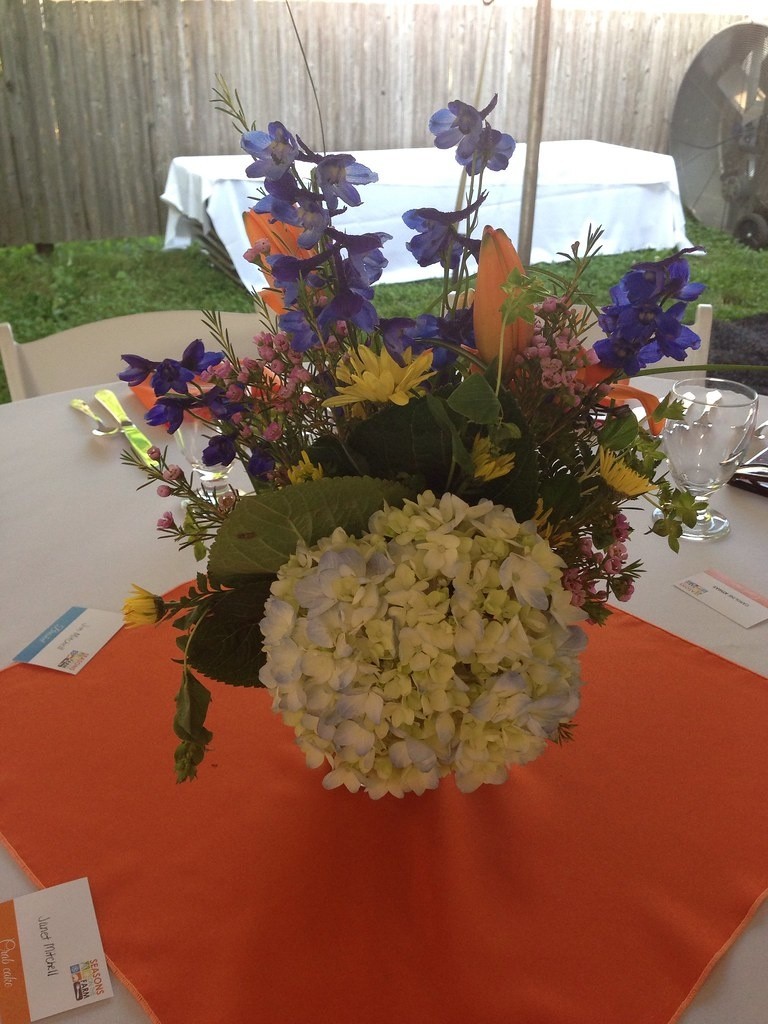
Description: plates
590,377,684,421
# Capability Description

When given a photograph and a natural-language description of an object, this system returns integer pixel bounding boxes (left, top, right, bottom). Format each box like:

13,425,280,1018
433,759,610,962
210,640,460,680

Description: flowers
117,0,715,802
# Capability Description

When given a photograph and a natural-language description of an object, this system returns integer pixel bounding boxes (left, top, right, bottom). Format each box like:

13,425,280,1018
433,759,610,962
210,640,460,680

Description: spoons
70,398,122,440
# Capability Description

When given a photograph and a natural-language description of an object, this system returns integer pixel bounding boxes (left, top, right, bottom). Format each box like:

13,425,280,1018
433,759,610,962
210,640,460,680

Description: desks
0,372,768,1024
159,137,707,297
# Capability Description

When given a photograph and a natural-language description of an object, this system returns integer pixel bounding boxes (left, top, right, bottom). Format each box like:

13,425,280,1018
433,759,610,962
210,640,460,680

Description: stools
0,310,283,403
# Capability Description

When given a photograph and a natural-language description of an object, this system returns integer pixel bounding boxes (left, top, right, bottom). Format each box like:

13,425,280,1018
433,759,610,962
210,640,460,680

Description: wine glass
646,376,758,544
169,404,248,510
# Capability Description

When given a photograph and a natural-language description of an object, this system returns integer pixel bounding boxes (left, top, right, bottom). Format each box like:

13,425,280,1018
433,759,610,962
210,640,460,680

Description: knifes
94,387,159,469
